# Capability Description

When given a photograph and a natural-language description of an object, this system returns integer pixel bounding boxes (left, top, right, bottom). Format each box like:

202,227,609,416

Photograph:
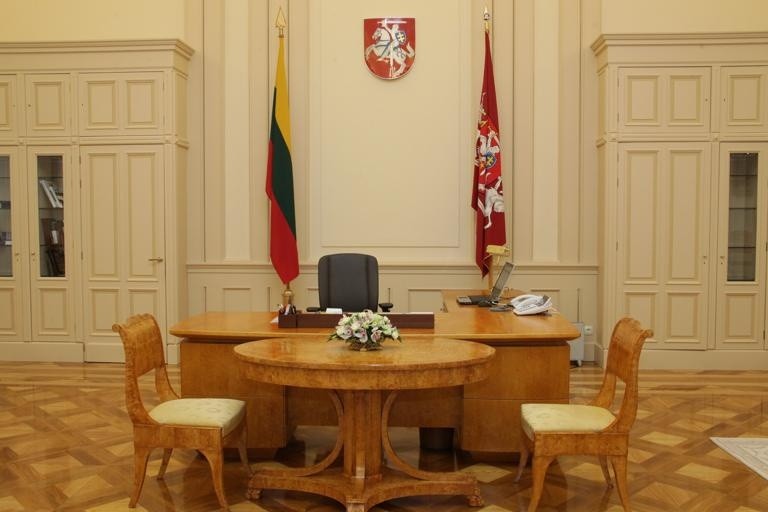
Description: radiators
569,323,587,365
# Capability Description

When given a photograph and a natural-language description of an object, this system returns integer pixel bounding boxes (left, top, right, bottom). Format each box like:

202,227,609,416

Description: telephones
510,294,554,315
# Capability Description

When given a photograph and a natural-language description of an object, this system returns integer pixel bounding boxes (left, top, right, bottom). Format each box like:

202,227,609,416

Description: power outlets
586,326,593,337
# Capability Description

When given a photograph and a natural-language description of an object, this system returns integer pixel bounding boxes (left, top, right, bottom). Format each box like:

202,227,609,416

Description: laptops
456,261,516,303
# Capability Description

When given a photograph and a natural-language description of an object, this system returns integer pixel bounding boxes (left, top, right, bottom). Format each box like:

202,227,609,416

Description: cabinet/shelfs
0,38,195,364
589,30,767,373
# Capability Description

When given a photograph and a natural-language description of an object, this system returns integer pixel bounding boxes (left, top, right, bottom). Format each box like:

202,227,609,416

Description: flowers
326,312,402,346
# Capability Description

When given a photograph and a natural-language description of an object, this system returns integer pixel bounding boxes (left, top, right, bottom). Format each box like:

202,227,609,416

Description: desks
235,337,497,512
169,289,580,464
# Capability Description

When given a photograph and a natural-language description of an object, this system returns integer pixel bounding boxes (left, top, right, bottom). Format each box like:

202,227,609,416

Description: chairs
111,314,254,511
513,317,654,511
317,254,379,312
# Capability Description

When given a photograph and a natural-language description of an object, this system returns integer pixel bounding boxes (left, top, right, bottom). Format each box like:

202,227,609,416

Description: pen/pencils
277,304,284,314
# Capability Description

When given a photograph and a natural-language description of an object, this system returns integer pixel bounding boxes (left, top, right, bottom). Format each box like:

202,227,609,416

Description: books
38,178,63,277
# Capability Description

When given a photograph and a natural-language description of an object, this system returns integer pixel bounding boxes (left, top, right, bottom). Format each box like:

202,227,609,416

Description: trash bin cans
566,322,585,367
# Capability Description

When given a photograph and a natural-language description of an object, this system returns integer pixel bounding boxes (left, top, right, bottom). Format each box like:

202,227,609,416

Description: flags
468,4,509,281
264,6,300,290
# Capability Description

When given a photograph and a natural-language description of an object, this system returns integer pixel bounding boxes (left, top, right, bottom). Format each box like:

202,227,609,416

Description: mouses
478,300,492,307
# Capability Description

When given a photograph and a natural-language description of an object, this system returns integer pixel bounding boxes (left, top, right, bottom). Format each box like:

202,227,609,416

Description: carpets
708,437,768,481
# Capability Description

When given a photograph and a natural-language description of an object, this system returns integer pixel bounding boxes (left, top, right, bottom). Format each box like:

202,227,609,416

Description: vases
347,340,385,351
